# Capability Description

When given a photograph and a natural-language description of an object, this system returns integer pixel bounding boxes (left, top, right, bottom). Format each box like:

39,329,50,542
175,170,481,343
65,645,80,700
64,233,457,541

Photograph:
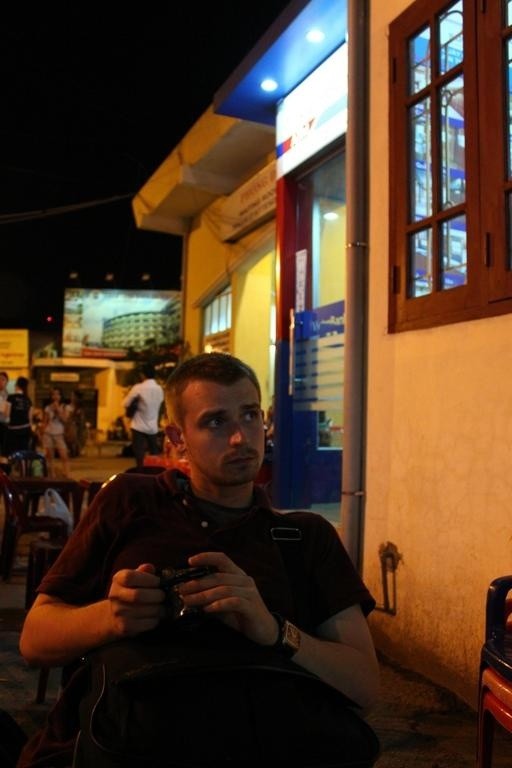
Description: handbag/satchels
76,627,382,767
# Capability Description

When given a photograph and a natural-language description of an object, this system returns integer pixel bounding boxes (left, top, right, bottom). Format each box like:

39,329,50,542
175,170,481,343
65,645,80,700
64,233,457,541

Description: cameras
152,547,209,613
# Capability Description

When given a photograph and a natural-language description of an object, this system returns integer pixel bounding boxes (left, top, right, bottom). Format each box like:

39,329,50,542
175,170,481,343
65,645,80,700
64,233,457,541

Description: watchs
264,616,303,662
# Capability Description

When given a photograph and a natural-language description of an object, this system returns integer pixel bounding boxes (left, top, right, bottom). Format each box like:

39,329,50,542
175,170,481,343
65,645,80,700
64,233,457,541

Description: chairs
0,423,512,768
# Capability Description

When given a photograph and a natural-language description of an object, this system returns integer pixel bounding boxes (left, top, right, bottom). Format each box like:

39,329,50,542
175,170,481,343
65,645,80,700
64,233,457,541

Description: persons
122,364,165,470
1,371,75,479
263,407,274,464
19,351,380,767
